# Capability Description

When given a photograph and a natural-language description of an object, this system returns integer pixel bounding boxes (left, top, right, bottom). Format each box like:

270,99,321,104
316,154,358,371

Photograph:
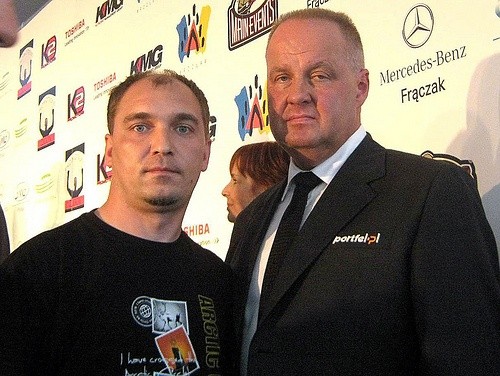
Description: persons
226,7,500,376
1,68,241,376
220,141,290,223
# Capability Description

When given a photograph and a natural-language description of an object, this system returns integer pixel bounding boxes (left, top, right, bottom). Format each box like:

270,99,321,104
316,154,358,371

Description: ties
257,170,325,329
